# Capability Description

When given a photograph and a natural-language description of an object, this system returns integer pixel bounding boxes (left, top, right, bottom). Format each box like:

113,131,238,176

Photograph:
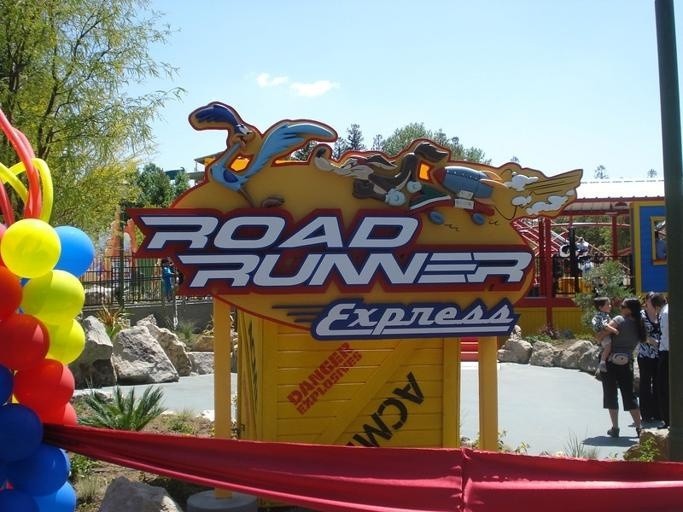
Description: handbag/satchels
608,351,630,366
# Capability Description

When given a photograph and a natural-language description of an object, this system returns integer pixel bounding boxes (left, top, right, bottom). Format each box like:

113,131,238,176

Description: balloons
0,106,96,511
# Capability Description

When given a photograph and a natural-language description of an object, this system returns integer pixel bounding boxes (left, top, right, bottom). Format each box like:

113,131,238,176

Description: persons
158,258,178,303
639,290,661,423
652,293,669,429
592,297,646,438
591,295,619,374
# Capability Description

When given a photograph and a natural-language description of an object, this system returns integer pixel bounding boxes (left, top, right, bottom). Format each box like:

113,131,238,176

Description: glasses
619,304,628,310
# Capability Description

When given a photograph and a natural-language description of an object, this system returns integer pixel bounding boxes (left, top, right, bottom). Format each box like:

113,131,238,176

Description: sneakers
607,427,620,438
636,417,669,437
